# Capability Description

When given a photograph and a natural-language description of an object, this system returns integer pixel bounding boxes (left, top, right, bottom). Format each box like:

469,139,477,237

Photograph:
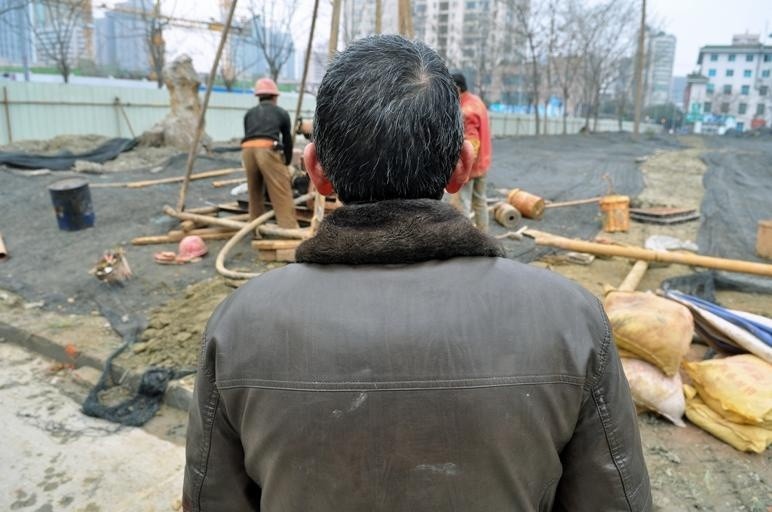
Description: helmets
254,79,281,97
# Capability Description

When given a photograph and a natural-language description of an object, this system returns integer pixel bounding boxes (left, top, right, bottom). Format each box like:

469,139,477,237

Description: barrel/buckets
508,186,545,219
492,202,521,229
756,218,772,260
599,195,629,234
48,179,93,231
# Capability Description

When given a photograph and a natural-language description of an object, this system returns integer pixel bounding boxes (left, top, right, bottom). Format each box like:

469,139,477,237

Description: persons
450,73,491,236
181,33,656,512
241,77,302,240
